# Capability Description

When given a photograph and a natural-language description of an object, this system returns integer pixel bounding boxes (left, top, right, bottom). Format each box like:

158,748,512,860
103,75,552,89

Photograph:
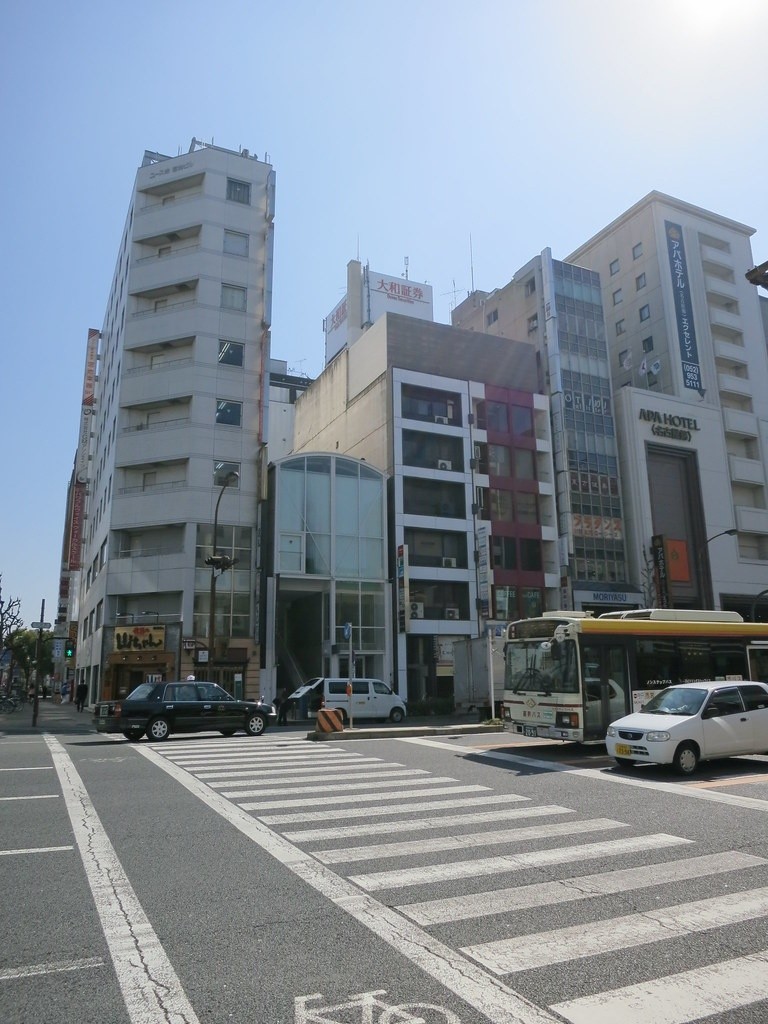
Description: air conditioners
440,557,457,568
443,608,461,620
438,460,452,471
434,415,449,425
439,501,456,516
407,601,425,619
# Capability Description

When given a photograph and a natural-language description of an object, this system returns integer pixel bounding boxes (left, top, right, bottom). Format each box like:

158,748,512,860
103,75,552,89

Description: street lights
26,656,30,694
696,528,738,610
208,471,240,683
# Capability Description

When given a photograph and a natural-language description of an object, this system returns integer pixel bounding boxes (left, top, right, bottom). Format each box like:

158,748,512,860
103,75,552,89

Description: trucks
452,635,540,713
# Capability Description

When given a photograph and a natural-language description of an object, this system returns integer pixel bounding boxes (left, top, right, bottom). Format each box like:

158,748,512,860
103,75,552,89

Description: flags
623,348,663,376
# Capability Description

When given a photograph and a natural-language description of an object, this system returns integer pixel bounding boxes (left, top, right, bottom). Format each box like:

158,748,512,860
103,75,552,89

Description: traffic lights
65,639,75,658
205,556,230,570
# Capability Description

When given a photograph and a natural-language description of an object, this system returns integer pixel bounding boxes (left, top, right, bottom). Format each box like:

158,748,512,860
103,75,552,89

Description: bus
501,608,768,744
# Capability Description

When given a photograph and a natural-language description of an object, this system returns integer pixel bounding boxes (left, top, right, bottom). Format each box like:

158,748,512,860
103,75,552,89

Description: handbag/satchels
74,697,79,704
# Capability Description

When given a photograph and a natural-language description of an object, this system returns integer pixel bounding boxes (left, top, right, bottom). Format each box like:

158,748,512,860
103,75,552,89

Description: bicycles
0,694,35,714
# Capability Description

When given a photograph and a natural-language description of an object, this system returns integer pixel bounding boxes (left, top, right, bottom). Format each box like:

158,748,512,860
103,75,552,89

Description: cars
92,682,278,740
606,682,768,776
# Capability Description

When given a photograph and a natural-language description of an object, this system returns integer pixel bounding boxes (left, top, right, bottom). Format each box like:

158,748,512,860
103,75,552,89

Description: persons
60,682,69,704
42,686,48,700
569,646,607,690
194,688,208,699
664,690,700,714
277,690,297,726
28,684,35,703
76,679,87,713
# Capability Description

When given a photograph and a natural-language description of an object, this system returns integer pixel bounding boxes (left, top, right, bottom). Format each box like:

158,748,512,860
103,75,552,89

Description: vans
288,677,406,724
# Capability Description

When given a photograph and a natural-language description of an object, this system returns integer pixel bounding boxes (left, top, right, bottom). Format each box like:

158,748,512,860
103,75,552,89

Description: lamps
116,611,135,624
140,610,159,622
